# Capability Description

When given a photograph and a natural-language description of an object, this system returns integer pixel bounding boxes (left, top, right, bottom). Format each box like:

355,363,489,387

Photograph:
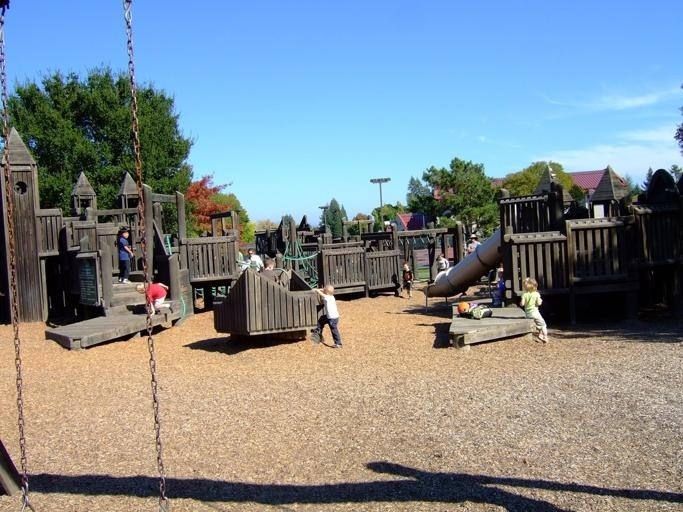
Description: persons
491,270,505,305
519,276,549,343
135,283,173,316
311,285,344,348
402,260,415,300
465,234,481,256
243,248,263,272
436,254,449,273
257,259,278,283
238,249,243,262
117,227,133,283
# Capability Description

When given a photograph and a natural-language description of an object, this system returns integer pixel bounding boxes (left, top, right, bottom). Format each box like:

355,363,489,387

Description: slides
423,225,501,297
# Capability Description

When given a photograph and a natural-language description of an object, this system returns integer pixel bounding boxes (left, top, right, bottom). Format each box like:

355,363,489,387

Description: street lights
370,176,393,234
317,205,328,226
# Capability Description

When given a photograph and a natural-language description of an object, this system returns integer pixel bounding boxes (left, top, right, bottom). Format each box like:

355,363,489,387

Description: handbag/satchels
460,303,492,320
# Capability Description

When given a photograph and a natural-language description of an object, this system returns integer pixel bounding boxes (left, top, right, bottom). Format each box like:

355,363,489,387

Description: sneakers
331,343,342,348
169,303,175,313
538,334,549,344
118,277,131,284
311,328,322,335
